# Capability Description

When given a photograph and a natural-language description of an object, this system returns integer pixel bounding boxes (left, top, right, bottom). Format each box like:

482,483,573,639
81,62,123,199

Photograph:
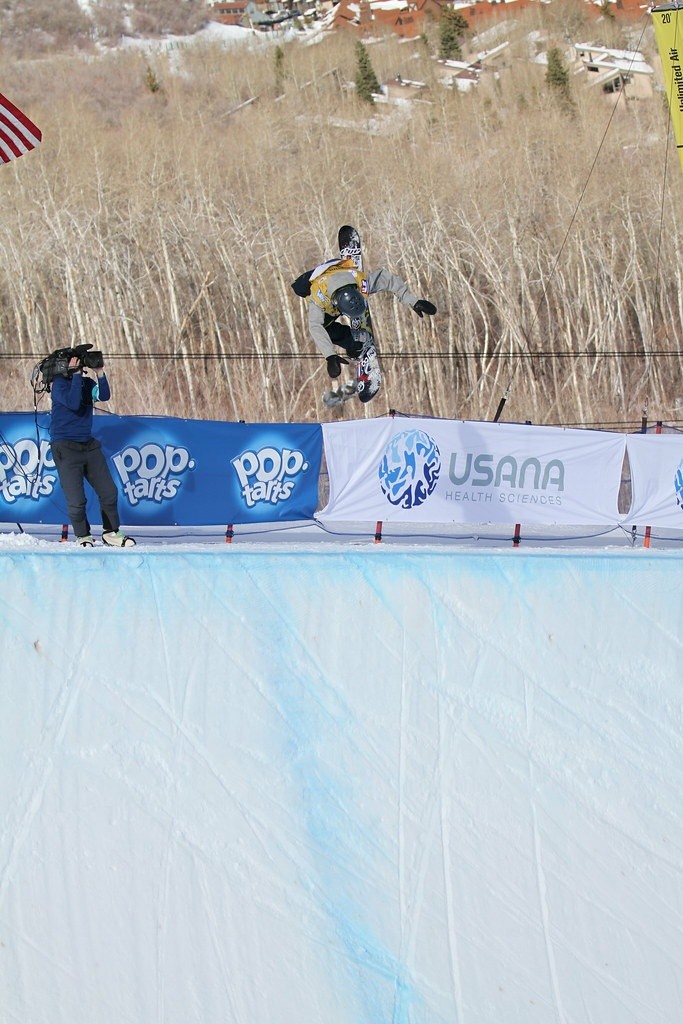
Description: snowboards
338,225,382,403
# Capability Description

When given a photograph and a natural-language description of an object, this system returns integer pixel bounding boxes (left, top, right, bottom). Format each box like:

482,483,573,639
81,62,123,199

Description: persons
51,357,136,547
306,258,437,377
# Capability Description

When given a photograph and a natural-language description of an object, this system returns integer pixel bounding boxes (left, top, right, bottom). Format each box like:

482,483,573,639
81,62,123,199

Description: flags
0,93,41,165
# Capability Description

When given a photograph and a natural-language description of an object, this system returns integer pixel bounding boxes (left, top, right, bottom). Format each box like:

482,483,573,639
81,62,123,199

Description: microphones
74,344,93,352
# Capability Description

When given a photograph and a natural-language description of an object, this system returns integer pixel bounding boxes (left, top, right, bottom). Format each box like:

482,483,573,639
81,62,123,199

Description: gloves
326,355,350,378
413,300,438,319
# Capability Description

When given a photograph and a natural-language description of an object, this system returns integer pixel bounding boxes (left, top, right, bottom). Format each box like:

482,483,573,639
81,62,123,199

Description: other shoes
345,342,369,360
76,535,94,548
101,531,138,547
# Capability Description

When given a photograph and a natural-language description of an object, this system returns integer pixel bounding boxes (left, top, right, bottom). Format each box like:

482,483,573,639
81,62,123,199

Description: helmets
337,288,366,316
